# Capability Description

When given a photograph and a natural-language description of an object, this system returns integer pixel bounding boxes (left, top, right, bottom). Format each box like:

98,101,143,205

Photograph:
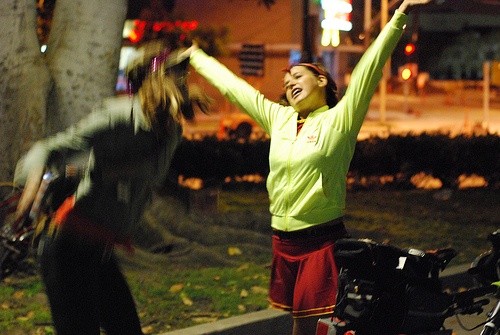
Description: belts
272,222,346,238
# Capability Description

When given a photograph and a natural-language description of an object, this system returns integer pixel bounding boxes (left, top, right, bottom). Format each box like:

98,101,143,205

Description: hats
281,61,338,95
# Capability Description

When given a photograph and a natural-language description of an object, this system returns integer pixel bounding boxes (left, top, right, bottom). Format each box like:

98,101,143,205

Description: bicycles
312,228,500,335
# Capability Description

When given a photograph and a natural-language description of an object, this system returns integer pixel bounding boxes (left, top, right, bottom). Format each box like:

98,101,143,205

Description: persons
8,36,215,335
166,0,415,333
